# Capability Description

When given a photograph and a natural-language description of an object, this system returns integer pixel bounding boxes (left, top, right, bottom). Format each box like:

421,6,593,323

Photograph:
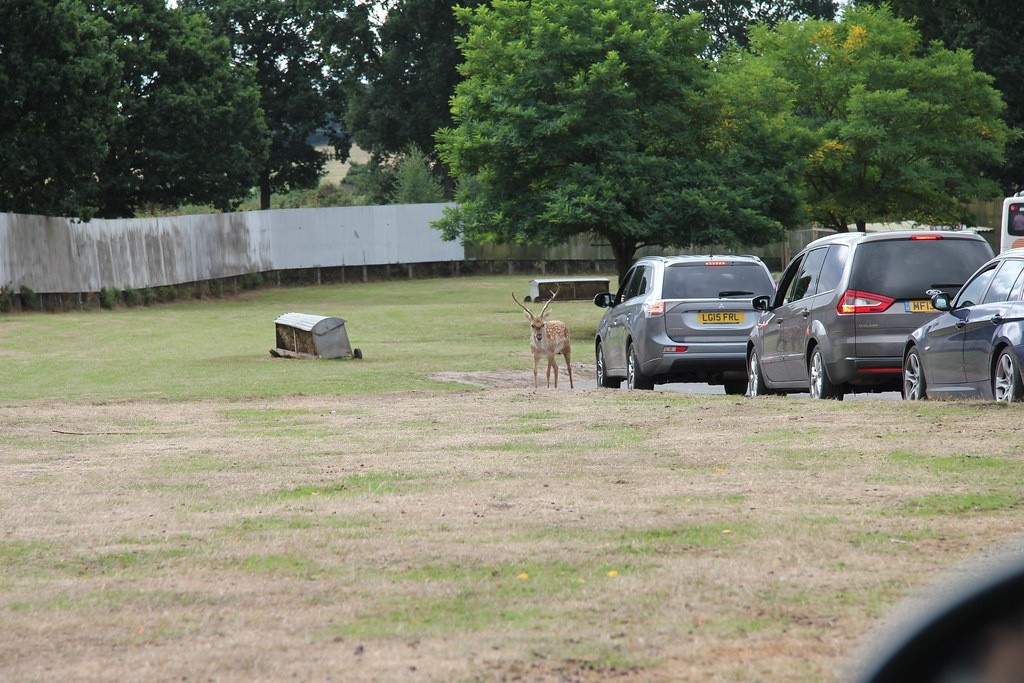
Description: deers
511,284,574,390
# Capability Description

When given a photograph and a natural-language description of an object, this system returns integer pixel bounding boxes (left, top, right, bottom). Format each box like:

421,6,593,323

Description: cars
901,247,1024,404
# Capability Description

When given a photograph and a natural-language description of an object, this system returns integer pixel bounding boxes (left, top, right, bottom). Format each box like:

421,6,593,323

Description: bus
1000,190,1024,253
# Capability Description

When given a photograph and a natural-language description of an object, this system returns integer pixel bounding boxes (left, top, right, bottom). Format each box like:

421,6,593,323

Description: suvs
745,230,996,402
593,255,785,396
